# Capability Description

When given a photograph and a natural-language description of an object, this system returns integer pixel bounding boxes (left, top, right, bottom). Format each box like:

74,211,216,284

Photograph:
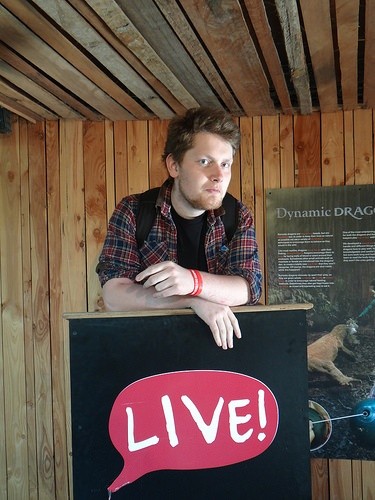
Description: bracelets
189,268,203,296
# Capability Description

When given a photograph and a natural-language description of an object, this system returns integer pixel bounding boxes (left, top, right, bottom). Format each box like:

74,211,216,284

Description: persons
95,107,264,350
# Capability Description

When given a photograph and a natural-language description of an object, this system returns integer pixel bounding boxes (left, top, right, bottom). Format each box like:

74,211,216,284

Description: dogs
306,324,364,385
